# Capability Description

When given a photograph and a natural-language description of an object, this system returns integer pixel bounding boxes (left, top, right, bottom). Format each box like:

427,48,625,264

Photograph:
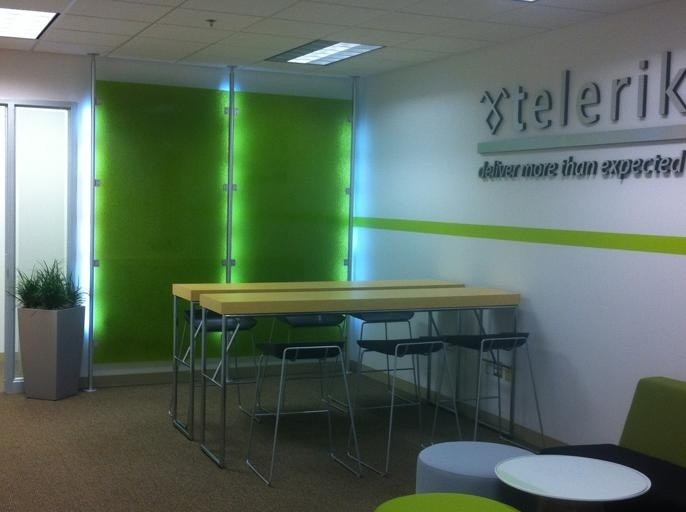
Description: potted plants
5,260,90,401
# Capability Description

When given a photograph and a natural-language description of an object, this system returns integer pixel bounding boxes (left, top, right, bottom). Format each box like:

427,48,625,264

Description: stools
373,439,539,512
169,299,264,425
345,337,462,478
326,311,424,411
419,332,544,442
245,342,362,483
255,314,352,418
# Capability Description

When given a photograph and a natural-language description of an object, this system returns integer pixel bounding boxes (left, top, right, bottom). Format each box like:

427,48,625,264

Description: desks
198,286,520,470
169,280,471,442
